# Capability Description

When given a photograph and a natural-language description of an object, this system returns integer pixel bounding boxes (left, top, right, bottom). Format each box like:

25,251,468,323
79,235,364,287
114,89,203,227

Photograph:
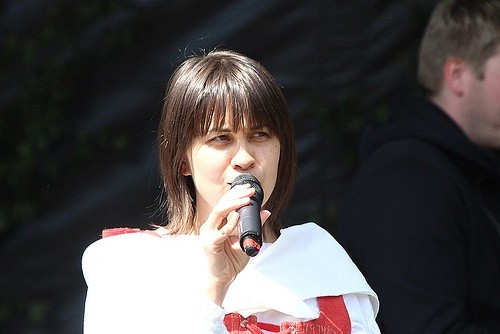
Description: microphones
230,173,264,257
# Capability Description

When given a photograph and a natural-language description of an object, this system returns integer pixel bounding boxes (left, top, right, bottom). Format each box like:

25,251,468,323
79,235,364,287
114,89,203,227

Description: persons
334,0,500,334
81,49,382,333
